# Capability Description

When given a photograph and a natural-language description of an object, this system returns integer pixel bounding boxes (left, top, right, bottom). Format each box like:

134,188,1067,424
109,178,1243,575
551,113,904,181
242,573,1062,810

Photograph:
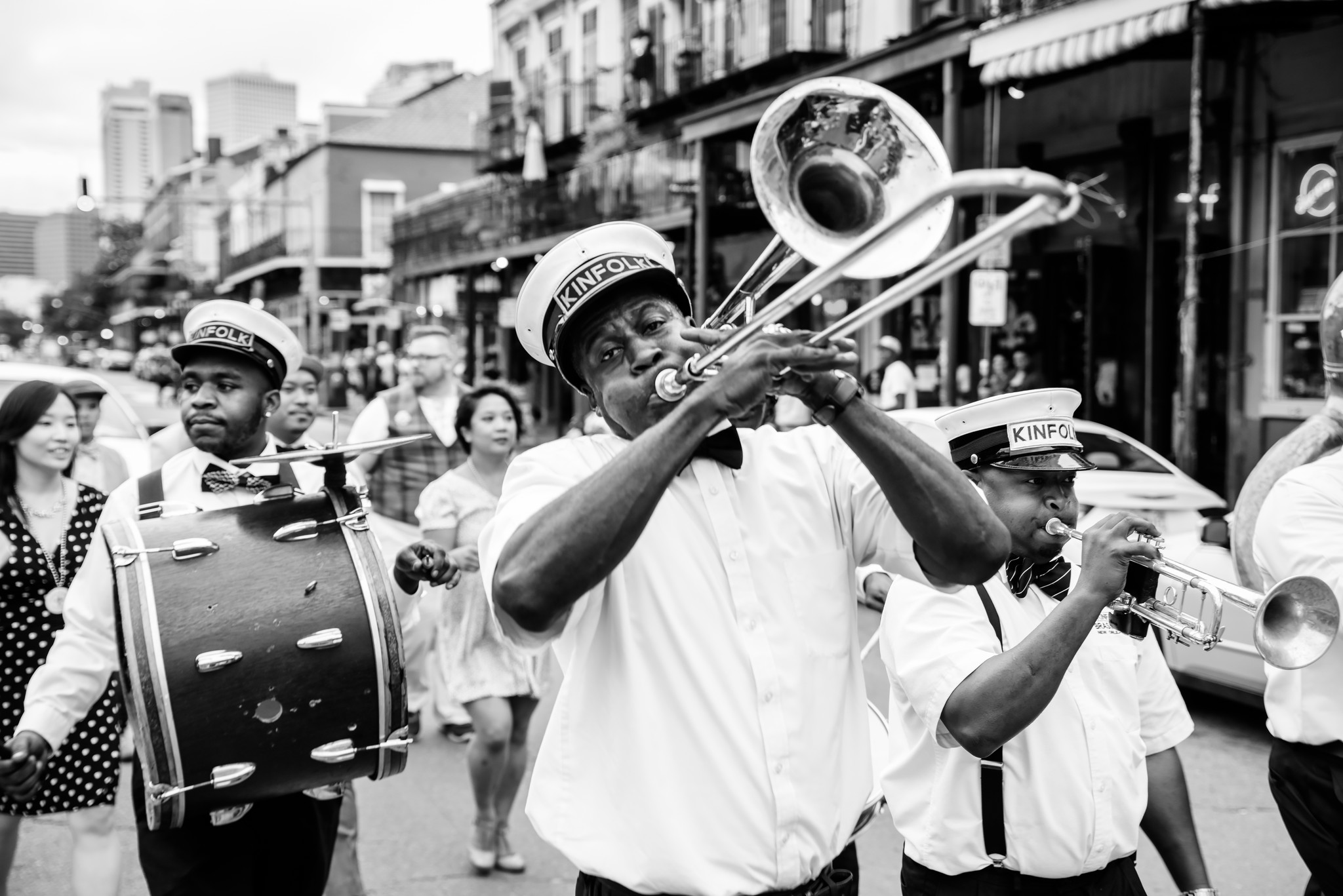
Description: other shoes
497,845,526,871
468,829,496,869
445,721,475,744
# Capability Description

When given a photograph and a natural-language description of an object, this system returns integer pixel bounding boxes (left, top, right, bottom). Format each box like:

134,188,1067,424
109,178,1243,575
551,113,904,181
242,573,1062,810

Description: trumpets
1047,516,1342,671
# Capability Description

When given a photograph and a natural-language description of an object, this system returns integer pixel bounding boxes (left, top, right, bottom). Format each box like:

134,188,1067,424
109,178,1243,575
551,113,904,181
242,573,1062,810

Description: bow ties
204,466,270,494
276,445,307,454
673,427,744,479
1004,549,1072,601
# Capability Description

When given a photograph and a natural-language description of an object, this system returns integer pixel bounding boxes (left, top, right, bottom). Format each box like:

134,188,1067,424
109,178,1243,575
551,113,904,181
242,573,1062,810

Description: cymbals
228,433,433,468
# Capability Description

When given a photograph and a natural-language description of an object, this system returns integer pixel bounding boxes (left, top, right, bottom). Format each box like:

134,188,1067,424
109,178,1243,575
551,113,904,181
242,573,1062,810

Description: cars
886,406,1233,549
0,361,159,500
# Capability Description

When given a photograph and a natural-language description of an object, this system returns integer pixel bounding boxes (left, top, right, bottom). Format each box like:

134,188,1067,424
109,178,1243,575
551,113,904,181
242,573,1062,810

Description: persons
2,219,1343,896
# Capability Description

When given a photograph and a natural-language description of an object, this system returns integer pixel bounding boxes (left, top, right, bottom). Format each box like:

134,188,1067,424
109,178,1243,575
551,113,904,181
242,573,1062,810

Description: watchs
808,368,865,427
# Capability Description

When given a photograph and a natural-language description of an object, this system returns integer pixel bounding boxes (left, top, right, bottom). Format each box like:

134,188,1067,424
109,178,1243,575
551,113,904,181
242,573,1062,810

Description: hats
301,356,324,383
935,387,1100,472
172,296,303,389
514,220,697,397
63,381,108,398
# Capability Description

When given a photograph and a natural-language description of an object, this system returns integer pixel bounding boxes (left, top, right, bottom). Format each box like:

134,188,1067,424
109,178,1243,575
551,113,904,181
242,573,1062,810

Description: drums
100,484,409,832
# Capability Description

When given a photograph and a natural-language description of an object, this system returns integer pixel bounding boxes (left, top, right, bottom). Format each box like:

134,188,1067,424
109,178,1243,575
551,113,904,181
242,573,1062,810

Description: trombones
655,75,1084,408
